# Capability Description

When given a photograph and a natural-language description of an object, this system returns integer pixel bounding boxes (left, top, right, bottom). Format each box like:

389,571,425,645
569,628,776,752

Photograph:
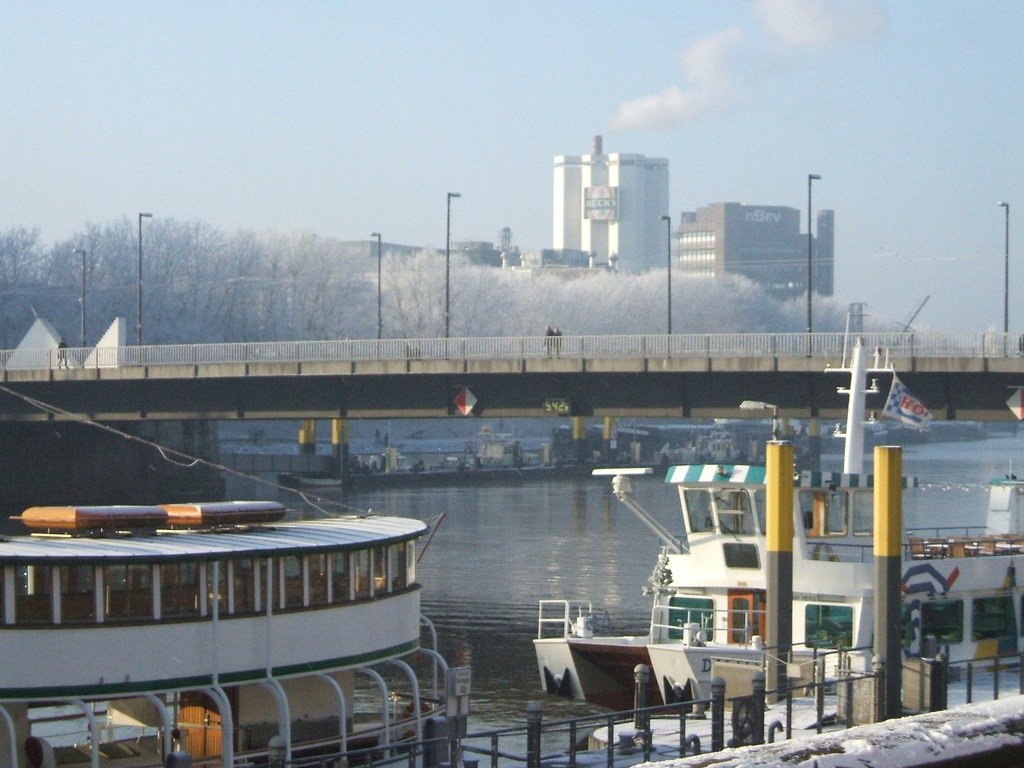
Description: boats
0,493,456,768
530,308,1024,714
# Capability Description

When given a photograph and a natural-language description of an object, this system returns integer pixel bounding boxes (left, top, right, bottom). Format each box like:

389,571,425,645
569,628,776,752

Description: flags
882,374,933,432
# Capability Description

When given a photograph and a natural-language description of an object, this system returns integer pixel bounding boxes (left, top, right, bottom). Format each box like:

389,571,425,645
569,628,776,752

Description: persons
553,327,562,359
58,338,70,369
545,325,553,359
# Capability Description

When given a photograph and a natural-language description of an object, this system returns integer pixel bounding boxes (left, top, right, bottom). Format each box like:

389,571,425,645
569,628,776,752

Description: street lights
369,232,382,359
73,248,87,368
807,173,823,357
995,200,1010,359
444,192,462,360
658,214,673,360
136,211,154,366
